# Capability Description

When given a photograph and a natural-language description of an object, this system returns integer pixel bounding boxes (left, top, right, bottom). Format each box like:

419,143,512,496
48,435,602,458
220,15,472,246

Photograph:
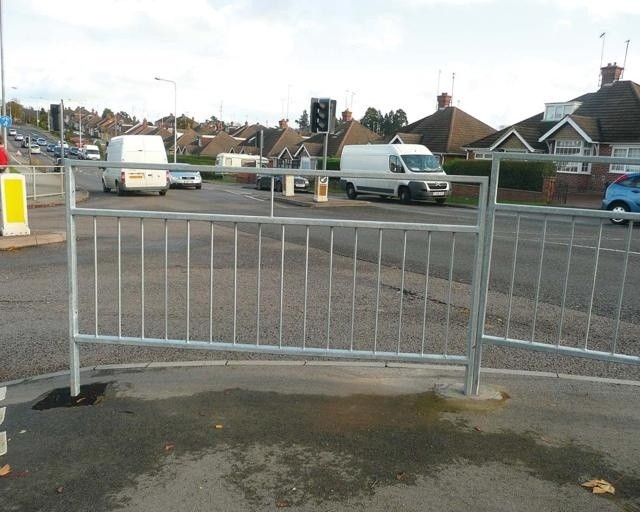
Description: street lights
155,78,176,163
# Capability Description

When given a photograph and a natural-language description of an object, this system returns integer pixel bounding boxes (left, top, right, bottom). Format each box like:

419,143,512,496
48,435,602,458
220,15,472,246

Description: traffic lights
310,97,336,134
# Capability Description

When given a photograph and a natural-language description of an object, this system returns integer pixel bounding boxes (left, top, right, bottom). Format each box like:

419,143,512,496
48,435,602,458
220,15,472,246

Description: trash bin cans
282,175,295,197
543,177,558,204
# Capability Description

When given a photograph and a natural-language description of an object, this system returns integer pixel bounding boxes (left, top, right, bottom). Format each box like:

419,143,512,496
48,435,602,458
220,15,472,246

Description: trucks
77,144,100,160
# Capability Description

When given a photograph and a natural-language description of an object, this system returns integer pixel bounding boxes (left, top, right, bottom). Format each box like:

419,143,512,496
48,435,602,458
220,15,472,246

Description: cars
47,140,78,172
256,174,310,193
9,129,46,153
167,163,202,189
601,172,640,224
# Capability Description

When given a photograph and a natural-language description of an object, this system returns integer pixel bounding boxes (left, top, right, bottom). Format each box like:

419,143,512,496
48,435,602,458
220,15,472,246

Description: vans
215,152,269,179
102,134,169,195
339,144,450,203
